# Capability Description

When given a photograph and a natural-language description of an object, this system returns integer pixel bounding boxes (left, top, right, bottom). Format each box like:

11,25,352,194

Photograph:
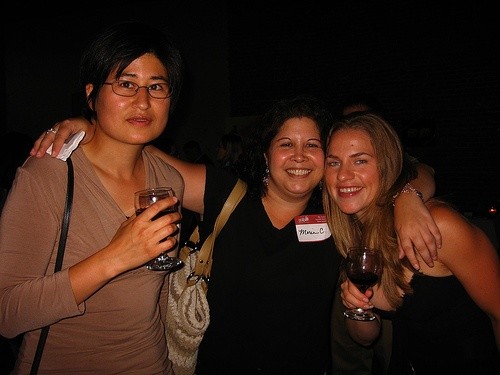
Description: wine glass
134,187,183,270
343,246,382,321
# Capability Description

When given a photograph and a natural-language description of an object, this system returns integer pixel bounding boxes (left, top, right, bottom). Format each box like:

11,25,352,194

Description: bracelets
390,183,423,206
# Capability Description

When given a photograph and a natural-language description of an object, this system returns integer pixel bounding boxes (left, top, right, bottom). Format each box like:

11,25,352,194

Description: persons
31,107,442,375
0,31,186,375
317,111,500,375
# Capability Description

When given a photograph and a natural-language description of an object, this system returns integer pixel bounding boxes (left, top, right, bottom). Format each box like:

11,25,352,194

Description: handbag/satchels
159,179,247,374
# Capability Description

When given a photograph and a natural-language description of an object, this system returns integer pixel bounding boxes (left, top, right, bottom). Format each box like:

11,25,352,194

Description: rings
45,127,57,136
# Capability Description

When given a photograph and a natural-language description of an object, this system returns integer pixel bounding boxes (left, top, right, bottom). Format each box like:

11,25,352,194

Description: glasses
104,79,174,99
